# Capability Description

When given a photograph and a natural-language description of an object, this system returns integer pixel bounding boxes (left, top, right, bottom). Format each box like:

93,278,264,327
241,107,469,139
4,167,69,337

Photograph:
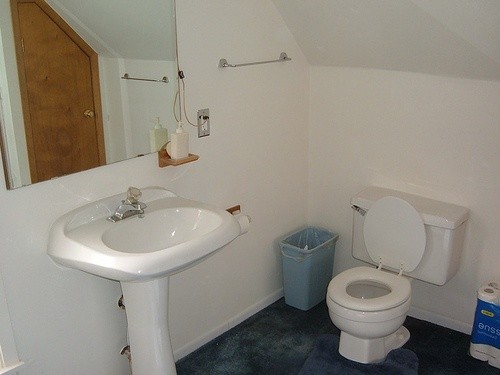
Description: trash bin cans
279,224,339,311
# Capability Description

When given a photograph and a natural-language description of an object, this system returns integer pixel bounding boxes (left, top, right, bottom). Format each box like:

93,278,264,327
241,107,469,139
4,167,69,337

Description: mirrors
0,0,183,190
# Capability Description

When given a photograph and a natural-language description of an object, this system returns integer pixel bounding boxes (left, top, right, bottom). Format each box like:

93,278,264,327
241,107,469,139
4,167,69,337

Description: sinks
46,186,241,374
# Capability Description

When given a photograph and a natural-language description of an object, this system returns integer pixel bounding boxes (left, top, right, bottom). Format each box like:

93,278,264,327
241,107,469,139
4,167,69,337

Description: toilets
326,185,470,365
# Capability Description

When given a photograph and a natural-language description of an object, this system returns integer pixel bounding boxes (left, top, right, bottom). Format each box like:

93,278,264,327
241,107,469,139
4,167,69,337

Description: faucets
108,186,148,222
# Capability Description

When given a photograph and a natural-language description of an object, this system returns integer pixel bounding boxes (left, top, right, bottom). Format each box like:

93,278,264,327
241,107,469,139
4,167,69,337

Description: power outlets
198,109,211,138
